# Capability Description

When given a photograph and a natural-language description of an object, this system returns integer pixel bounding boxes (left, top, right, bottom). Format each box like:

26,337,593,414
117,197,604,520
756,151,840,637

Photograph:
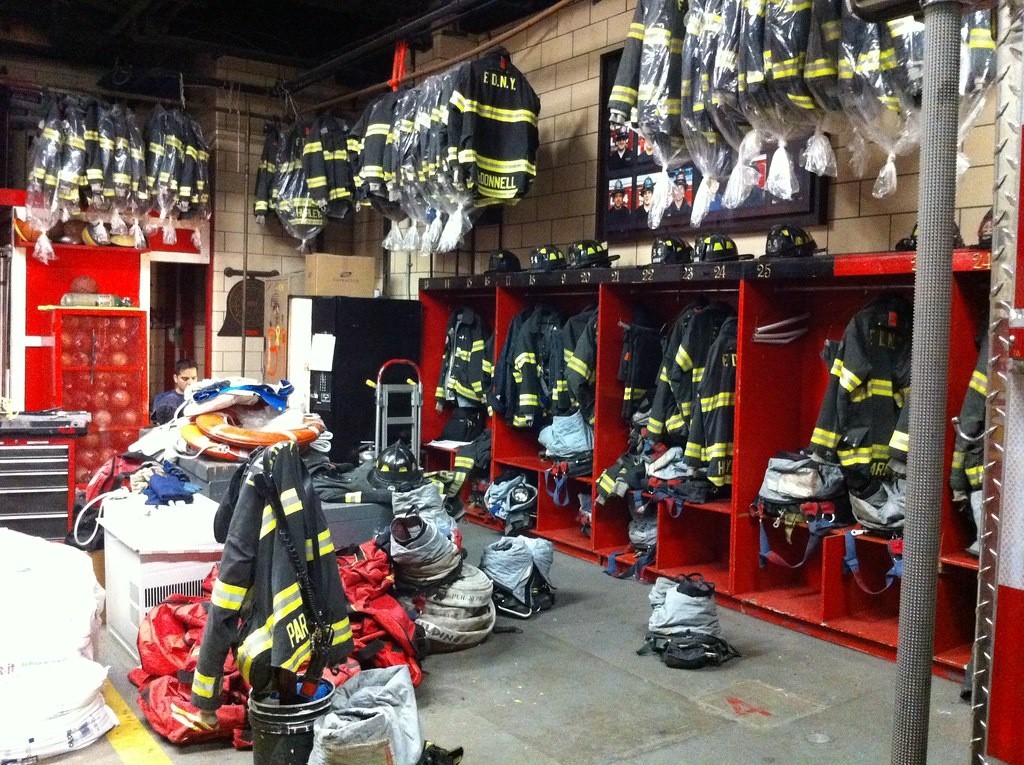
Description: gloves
145,460,203,506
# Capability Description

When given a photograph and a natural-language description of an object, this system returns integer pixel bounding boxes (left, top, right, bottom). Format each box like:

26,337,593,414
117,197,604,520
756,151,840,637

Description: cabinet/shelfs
0,436,77,541
414,250,995,687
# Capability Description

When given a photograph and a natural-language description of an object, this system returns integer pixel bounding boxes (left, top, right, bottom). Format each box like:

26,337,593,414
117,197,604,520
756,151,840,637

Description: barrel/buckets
247,674,336,765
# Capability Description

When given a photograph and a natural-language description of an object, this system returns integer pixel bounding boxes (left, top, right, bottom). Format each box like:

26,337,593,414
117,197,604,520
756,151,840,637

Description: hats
616,130,629,140
641,175,654,195
611,179,626,197
674,171,688,188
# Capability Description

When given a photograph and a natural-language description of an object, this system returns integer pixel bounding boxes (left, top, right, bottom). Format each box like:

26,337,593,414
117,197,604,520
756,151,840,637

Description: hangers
673,290,682,317
581,291,600,319
855,285,890,307
278,41,502,123
40,81,187,115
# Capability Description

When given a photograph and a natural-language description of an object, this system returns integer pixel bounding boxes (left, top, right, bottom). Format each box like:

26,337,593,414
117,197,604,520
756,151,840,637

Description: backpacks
441,406,486,442
86,449,147,507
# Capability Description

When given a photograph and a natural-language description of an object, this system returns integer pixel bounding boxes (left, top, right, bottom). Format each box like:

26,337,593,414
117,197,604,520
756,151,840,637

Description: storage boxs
304,253,375,298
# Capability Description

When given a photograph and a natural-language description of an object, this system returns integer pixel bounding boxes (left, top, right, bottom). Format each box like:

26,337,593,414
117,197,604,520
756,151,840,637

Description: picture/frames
595,48,829,245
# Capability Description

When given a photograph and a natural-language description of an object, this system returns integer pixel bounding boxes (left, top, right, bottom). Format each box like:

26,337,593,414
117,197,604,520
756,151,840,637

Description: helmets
651,233,696,263
484,249,522,272
694,232,740,262
366,439,423,492
568,239,621,269
759,222,819,258
529,244,567,272
895,218,966,251
977,206,994,248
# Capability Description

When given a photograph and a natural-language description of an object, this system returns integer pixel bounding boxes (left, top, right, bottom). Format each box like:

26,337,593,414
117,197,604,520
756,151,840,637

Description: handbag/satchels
64,491,106,552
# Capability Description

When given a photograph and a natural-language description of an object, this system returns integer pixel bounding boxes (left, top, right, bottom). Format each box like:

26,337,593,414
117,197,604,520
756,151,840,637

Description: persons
150,358,198,425
638,139,658,161
637,176,654,215
608,130,634,159
664,173,692,214
608,180,629,215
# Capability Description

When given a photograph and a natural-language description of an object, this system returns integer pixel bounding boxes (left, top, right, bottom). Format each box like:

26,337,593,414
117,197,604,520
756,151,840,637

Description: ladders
374,358,424,467
